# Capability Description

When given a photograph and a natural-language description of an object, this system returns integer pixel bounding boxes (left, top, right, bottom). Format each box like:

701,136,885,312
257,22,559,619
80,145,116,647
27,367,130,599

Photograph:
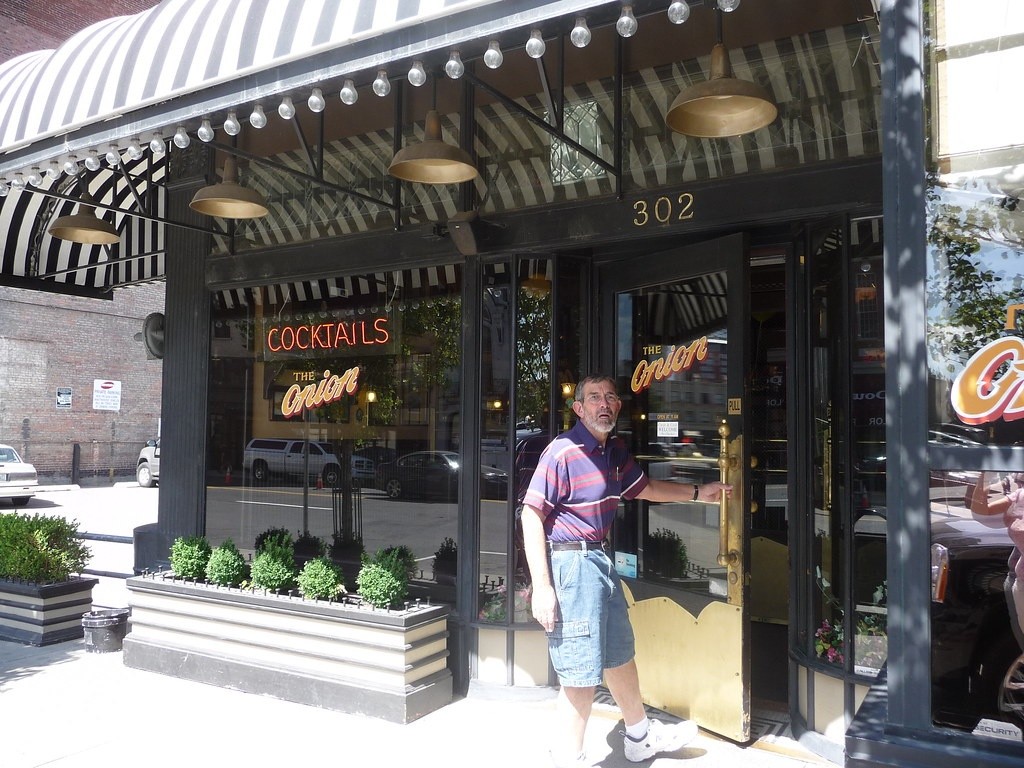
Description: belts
547,538,610,551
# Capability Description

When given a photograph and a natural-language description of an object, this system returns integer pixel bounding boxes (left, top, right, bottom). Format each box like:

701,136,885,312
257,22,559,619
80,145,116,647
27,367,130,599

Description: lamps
387,58,477,190
47,168,120,245
664,0,776,141
189,136,269,218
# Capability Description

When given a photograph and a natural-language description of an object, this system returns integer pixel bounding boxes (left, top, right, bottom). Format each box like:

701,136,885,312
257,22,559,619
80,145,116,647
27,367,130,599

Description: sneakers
619,719,699,762
549,745,601,768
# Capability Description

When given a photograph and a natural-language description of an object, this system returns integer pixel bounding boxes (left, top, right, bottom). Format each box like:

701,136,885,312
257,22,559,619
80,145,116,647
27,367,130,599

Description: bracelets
692,485,698,501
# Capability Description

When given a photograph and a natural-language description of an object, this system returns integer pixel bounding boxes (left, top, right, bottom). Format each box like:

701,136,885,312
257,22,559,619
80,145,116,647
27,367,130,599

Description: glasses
584,394,620,404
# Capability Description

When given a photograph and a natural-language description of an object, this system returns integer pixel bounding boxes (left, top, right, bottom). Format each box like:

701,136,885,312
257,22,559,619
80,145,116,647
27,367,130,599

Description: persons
521,374,734,768
970,471,1024,632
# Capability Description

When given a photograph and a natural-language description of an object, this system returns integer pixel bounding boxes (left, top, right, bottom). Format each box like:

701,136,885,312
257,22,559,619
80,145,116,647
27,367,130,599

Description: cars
354,445,400,463
0,444,39,506
136,438,162,487
375,450,510,499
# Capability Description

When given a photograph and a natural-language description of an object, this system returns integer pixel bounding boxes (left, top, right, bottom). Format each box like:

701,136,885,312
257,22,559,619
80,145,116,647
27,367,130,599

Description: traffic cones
222,466,231,488
316,470,325,490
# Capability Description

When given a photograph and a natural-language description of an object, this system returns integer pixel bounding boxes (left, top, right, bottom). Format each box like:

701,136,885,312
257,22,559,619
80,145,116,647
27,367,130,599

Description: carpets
587,686,794,749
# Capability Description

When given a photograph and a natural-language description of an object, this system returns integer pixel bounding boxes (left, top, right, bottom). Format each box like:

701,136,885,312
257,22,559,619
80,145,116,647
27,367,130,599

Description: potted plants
0,511,100,648
254,526,504,614
647,528,711,596
123,528,452,726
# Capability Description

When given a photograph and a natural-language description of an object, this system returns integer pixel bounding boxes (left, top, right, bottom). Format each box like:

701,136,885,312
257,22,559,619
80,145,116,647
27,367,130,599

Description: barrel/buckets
80,608,129,652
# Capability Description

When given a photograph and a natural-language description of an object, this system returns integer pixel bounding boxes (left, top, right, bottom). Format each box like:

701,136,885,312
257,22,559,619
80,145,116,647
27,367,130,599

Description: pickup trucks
245,439,376,484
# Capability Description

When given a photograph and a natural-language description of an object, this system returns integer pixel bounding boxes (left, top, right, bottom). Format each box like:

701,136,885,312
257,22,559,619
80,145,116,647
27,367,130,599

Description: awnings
0,0,931,300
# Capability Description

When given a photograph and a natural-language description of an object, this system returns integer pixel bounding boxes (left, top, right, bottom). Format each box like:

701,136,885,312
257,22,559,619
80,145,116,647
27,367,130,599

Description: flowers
812,567,888,677
478,581,533,625
